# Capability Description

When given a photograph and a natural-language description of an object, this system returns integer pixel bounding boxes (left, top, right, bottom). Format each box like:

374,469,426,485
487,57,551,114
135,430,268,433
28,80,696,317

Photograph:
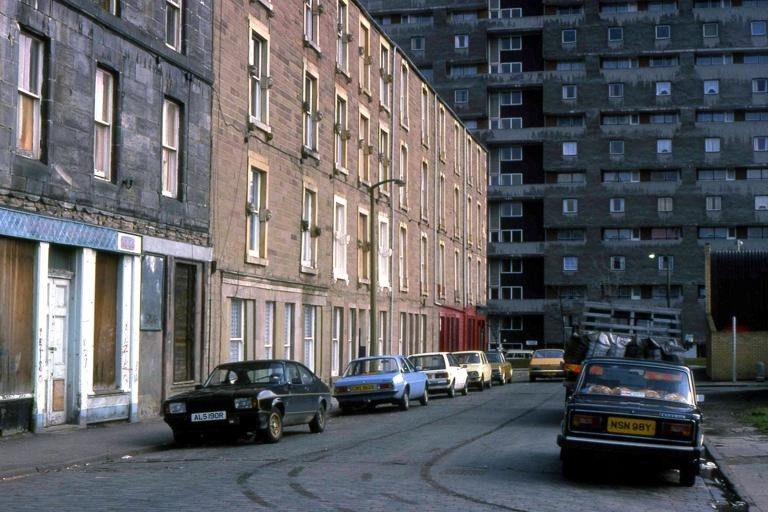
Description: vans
504,349,533,360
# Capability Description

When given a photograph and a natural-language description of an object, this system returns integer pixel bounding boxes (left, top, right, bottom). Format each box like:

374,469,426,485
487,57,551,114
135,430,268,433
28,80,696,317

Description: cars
528,349,565,383
452,351,491,391
162,359,331,444
332,355,428,411
401,352,468,397
556,357,704,488
484,351,512,386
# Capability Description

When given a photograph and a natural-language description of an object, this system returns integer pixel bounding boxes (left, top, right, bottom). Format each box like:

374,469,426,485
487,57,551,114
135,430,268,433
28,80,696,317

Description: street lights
368,178,405,372
647,251,672,309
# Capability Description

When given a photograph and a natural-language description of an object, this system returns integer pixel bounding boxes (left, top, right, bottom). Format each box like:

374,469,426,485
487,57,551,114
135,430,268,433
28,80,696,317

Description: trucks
559,323,684,407
